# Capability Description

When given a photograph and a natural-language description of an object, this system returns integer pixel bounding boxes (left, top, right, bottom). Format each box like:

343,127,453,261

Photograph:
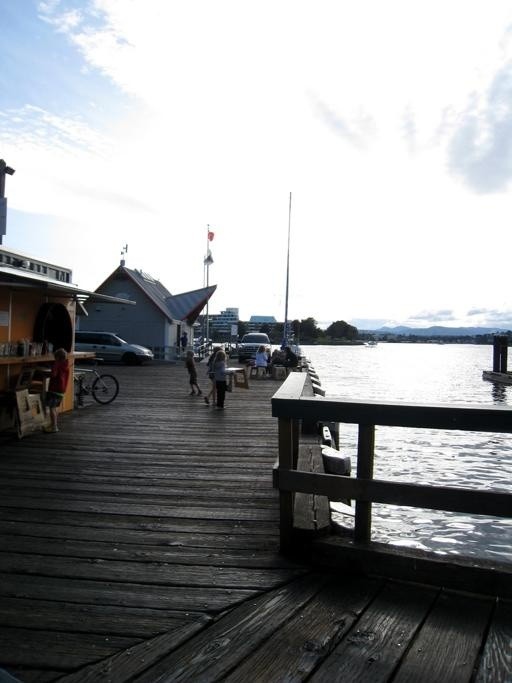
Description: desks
222,367,248,390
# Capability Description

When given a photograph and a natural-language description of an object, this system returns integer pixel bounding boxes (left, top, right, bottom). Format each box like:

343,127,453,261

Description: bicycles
74,357,120,405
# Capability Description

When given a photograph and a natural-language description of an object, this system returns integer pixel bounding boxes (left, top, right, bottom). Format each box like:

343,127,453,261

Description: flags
208,231,214,242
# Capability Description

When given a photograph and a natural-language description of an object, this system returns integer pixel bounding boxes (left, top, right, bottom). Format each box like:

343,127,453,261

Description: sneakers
204,396,210,403
212,402,226,410
44,427,58,433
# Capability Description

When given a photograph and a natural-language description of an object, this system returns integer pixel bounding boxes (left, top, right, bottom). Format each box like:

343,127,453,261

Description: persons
184,350,203,396
212,350,236,409
41,347,71,433
204,347,224,405
256,345,298,368
180,332,188,352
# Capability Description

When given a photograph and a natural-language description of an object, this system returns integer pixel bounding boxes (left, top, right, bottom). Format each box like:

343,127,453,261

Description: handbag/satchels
45,392,63,407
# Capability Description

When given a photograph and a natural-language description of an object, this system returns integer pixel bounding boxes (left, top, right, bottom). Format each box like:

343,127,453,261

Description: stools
250,366,266,378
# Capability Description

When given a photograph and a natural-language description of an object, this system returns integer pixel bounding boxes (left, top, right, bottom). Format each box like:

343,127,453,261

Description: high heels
190,390,202,396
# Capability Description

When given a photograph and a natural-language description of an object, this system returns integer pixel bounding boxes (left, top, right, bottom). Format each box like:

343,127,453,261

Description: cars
238,333,272,363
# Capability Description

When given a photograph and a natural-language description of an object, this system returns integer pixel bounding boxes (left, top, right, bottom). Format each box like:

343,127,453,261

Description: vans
75,331,155,365
